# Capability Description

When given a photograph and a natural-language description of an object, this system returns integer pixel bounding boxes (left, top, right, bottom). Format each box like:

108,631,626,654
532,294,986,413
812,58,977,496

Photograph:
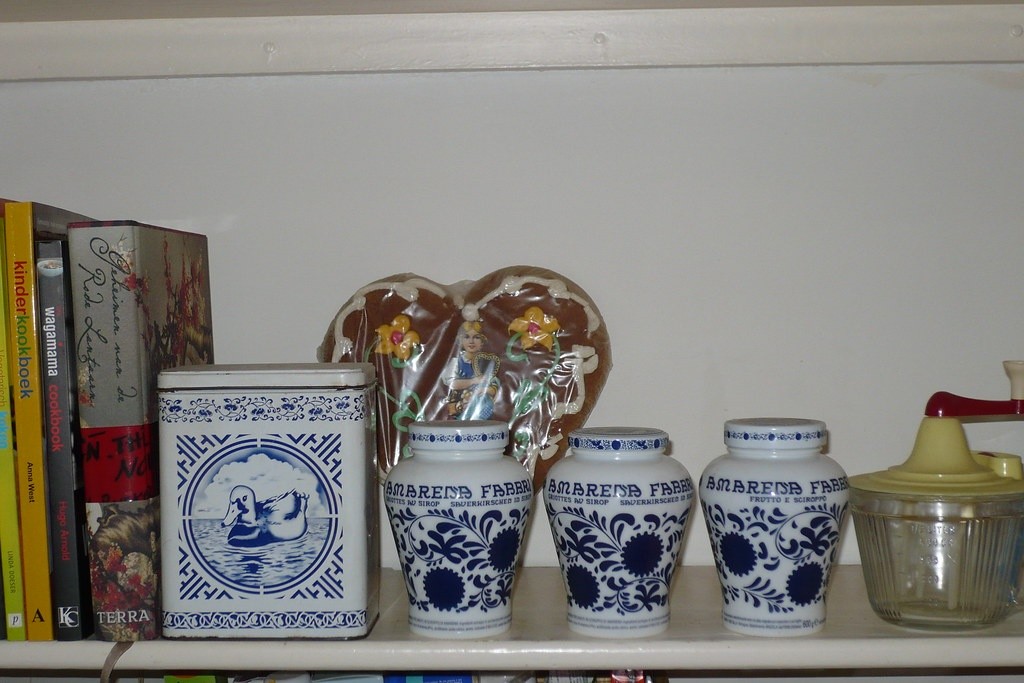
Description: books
0,197,28,641
66,218,222,641
36,237,96,642
3,208,94,644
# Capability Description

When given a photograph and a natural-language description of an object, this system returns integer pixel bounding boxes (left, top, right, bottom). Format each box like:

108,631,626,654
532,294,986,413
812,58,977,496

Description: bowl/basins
848,489,1024,632
